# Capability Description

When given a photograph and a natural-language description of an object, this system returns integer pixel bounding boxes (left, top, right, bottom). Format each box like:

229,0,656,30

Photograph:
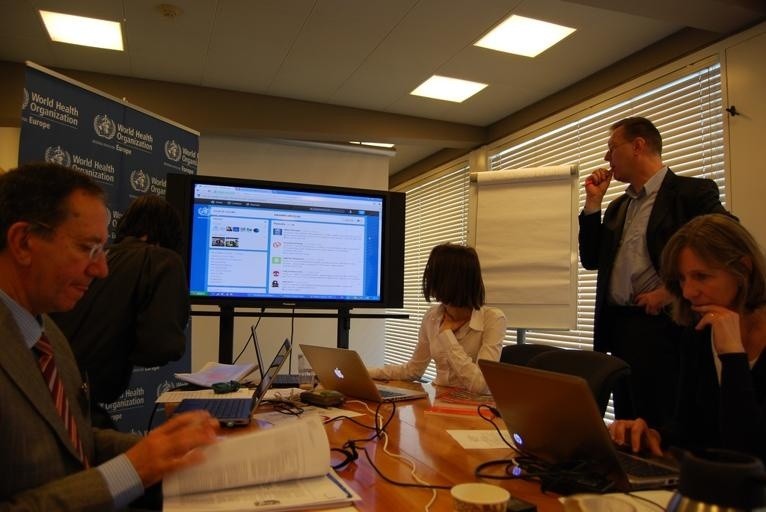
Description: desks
164,376,679,512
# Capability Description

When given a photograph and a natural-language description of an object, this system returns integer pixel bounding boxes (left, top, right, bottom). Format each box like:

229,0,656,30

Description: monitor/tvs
165,171,406,309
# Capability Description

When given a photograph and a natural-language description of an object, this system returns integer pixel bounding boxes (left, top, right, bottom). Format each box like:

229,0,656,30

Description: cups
298,355,316,389
451,483,511,512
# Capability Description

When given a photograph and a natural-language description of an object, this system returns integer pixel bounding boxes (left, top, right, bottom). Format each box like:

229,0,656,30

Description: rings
712,311,716,318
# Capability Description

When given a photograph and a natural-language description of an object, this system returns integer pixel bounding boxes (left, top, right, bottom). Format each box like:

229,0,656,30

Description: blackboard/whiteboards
463,164,581,335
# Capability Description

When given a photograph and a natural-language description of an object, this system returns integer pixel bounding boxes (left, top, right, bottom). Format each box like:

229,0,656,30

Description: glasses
607,137,641,154
23,220,113,262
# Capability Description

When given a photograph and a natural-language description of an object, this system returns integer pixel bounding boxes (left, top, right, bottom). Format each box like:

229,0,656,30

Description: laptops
299,343,429,403
170,338,293,425
477,358,681,493
251,324,317,388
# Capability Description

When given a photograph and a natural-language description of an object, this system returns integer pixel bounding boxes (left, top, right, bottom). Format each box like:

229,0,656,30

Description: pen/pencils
581,168,615,187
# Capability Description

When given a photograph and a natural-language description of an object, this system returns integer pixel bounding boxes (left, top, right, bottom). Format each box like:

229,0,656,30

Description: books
174,362,259,388
160,414,363,512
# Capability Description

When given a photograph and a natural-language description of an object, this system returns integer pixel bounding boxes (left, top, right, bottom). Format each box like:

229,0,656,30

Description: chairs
527,349,631,420
500,344,562,375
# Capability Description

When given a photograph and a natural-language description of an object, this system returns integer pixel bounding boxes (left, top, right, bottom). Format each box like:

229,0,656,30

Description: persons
606,214,766,457
0,162,221,512
578,117,740,420
365,242,507,395
47,195,190,432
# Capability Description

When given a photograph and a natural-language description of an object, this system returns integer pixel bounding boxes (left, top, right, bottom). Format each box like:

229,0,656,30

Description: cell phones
507,496,537,512
